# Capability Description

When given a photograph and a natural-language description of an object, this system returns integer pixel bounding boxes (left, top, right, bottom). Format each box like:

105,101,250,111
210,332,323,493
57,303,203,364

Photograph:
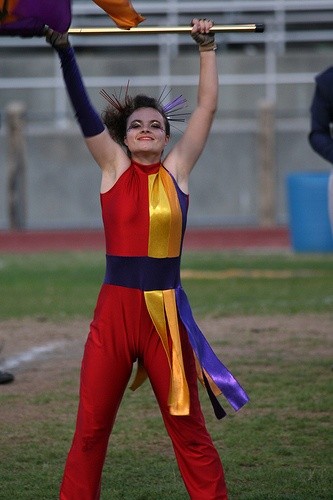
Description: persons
307,65,333,167
42,18,248,500
0,372,14,383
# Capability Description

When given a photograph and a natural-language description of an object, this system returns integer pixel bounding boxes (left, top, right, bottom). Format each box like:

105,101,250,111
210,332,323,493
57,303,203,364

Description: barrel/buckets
285,171,333,253
